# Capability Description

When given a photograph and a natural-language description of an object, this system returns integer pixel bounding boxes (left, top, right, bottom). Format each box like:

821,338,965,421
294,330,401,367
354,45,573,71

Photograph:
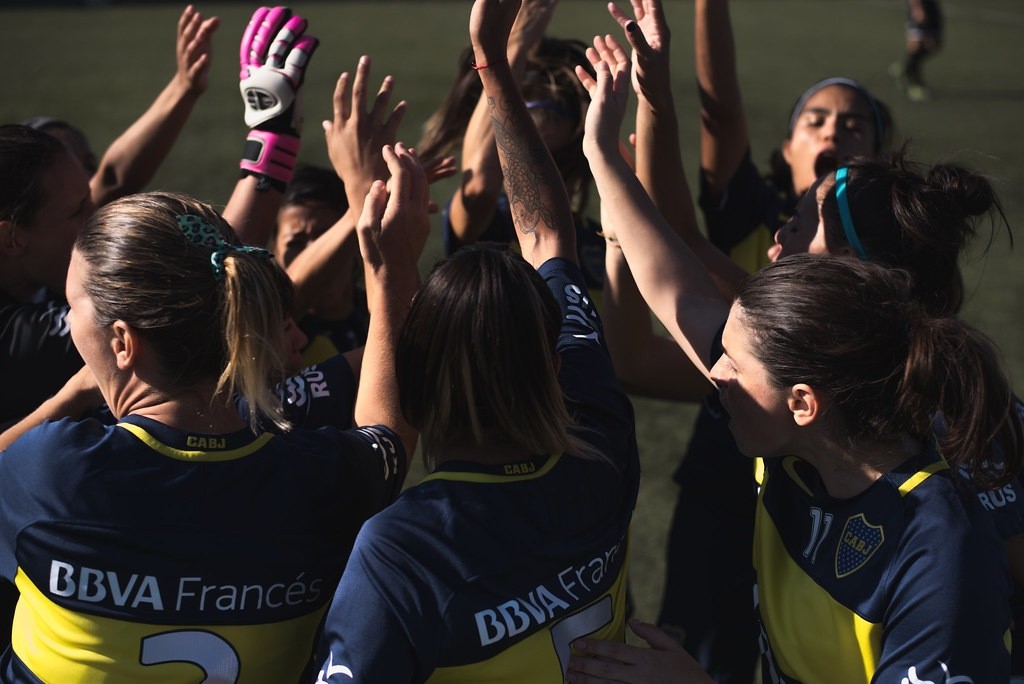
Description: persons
0,0,1023,684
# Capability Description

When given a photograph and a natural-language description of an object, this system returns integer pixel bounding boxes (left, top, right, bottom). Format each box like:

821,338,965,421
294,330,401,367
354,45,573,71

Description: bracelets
472,58,506,71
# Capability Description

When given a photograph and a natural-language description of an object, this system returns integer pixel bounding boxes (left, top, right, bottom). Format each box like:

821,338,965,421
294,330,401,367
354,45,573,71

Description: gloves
238,5,320,194
